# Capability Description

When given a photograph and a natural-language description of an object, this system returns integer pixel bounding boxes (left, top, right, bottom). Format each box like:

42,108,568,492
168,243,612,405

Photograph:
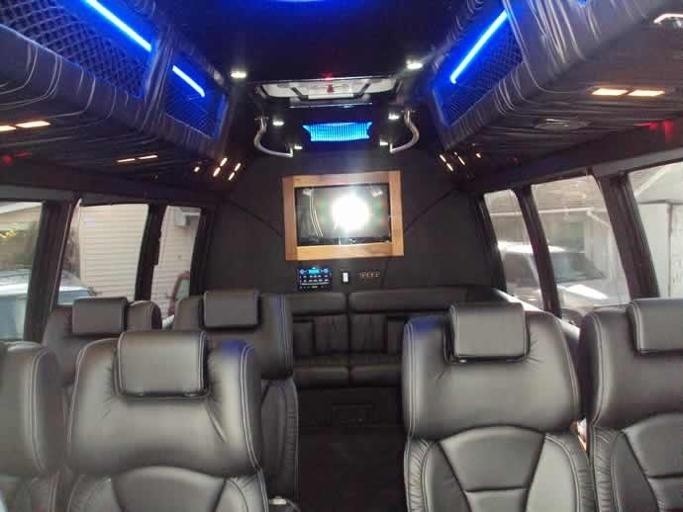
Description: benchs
291,287,491,397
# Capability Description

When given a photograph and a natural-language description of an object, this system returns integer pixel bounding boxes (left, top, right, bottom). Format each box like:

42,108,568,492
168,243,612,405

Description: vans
0,263,97,342
498,241,625,328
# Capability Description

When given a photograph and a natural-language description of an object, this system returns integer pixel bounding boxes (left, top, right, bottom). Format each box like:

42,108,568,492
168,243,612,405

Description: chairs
401,299,596,512
577,295,682,512
41,295,165,408
1,338,69,512
170,290,299,505
66,326,272,512
